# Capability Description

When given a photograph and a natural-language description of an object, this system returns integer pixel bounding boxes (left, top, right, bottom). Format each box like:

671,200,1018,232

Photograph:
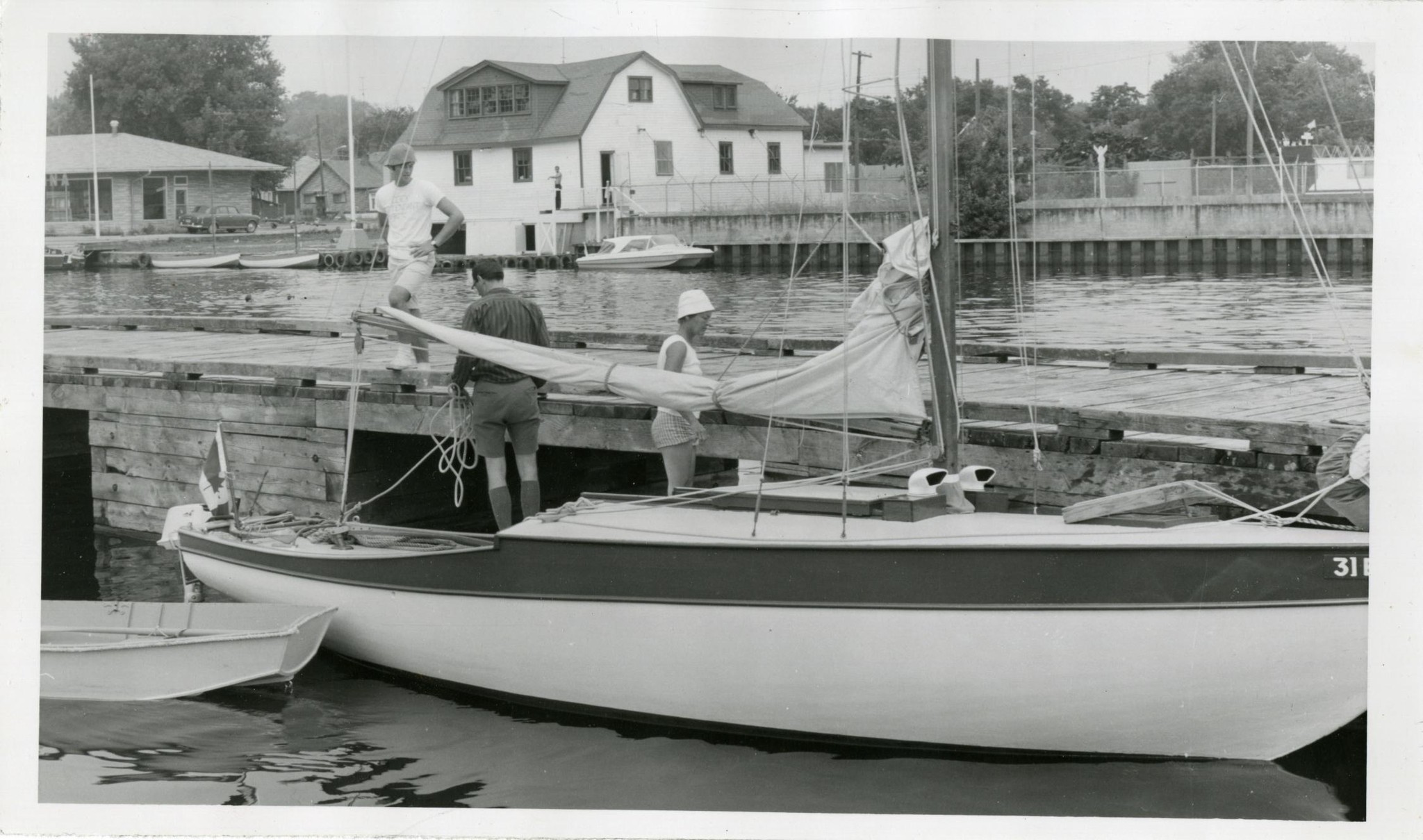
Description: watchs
429,239,439,249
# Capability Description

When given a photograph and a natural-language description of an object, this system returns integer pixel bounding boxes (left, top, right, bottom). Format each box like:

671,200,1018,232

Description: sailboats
159,38,1369,762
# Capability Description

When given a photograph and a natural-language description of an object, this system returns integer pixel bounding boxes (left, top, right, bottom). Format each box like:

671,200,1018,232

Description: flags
199,422,229,520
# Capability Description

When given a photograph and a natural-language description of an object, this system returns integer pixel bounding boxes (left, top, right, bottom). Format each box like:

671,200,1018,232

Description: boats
575,234,715,269
151,251,241,268
239,252,320,269
39,598,340,701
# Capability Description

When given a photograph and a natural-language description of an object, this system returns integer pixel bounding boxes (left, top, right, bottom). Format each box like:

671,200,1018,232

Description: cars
177,203,261,235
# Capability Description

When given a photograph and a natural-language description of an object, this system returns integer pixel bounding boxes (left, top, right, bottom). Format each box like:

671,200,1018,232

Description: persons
548,166,562,210
651,289,715,497
374,143,464,389
451,260,551,531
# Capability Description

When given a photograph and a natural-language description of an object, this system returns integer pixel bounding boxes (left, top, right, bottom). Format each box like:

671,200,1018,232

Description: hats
677,289,715,322
385,142,415,167
471,261,503,289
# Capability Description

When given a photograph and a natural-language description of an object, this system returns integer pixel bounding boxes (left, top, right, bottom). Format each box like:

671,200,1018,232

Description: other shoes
387,360,409,370
